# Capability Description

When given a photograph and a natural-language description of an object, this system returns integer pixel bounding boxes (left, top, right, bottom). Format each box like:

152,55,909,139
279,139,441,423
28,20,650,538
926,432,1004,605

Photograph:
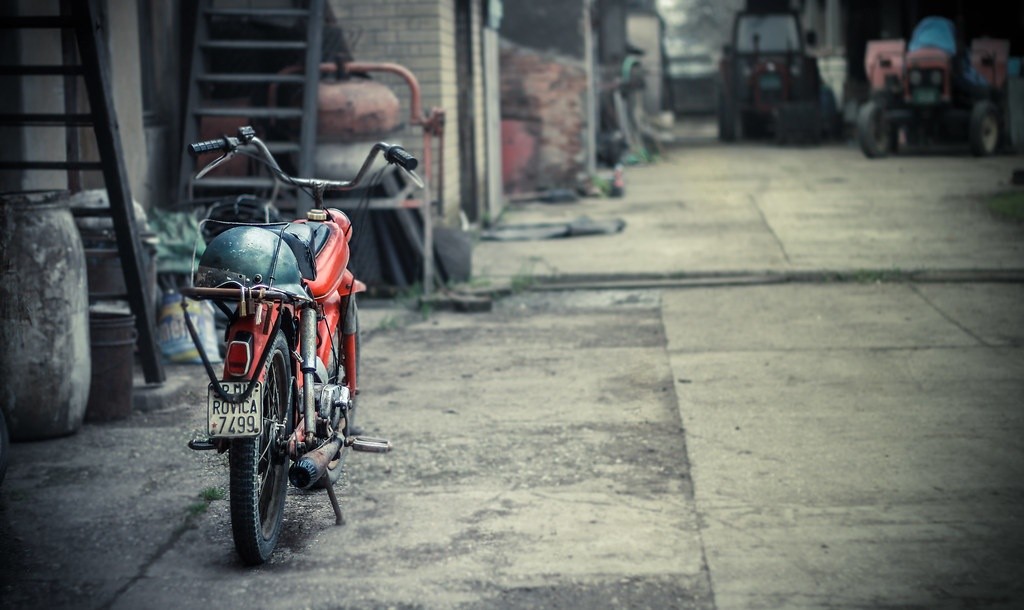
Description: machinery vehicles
713,4,830,150
853,11,1013,161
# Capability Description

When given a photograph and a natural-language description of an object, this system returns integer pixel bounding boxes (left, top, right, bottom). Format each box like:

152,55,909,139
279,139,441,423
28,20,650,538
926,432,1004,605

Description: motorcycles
178,126,427,562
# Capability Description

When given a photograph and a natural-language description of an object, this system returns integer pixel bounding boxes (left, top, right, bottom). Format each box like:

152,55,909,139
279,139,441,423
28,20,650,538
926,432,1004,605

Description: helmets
194,226,314,303
